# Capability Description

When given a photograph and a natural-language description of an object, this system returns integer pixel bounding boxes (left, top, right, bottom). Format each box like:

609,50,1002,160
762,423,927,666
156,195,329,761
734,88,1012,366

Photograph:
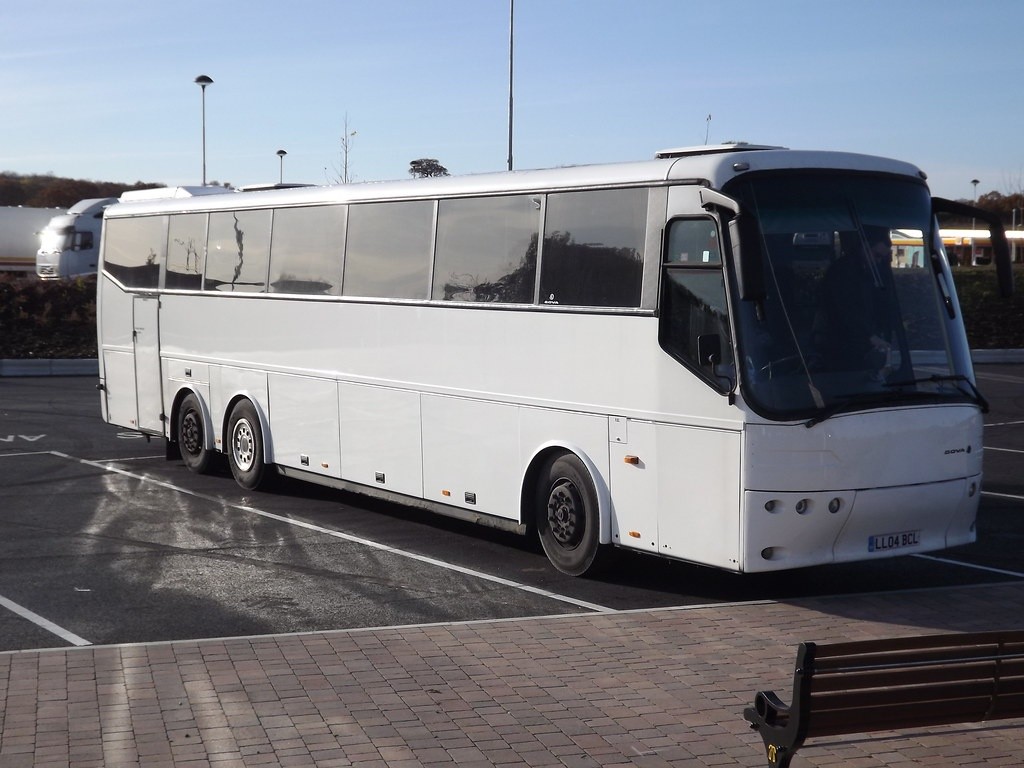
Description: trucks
33,197,117,284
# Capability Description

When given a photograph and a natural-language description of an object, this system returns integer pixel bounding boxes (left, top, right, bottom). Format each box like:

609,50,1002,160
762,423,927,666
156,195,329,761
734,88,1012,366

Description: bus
890,228,1024,268
96,142,1013,576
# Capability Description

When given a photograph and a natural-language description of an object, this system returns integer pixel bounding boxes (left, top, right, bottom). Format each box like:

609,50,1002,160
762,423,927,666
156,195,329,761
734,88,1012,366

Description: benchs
743,628,1024,768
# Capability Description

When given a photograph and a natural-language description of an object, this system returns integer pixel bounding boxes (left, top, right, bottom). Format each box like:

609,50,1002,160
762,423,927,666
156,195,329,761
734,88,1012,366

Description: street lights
971,180,979,229
1012,209,1016,230
277,150,287,183
194,75,214,186
